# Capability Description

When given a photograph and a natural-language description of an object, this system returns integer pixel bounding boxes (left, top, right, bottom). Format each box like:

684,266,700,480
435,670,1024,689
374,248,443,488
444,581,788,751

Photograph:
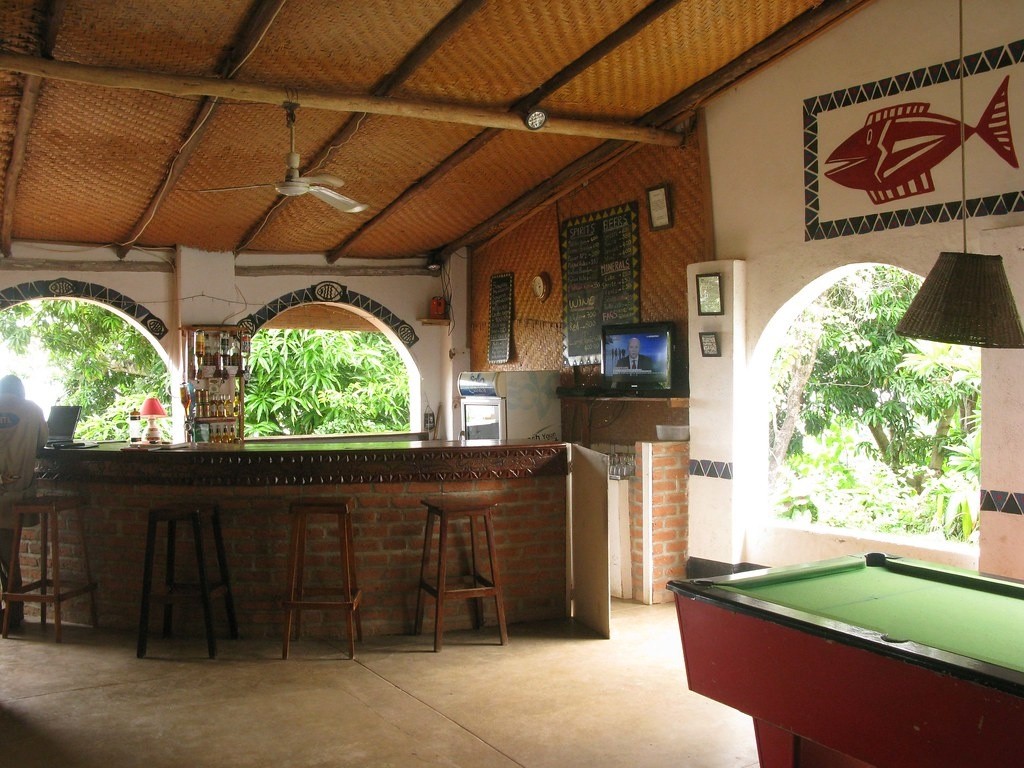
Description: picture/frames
697,272,725,316
698,332,722,357
644,180,673,232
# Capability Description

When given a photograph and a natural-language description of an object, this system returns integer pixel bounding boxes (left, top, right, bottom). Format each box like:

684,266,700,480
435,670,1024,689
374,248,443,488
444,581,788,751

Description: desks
662,552,1024,768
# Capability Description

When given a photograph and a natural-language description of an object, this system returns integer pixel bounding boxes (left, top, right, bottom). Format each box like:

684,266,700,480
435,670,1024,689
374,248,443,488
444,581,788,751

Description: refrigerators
457,371,561,440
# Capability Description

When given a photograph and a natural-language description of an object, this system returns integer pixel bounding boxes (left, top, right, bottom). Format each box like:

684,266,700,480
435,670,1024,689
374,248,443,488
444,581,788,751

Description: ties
632,360,636,369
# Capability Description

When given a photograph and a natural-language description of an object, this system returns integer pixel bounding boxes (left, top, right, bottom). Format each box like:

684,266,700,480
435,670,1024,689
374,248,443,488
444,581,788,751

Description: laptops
46,405,82,444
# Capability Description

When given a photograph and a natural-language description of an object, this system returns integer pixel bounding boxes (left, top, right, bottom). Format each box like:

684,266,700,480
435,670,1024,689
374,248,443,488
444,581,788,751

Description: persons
617,338,653,370
0,374,49,632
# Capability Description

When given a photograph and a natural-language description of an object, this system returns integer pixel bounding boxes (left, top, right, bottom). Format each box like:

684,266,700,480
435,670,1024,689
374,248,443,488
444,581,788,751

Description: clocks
531,276,550,302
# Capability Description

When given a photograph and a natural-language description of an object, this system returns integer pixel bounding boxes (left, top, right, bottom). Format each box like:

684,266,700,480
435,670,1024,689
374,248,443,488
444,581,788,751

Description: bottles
129,408,141,441
605,453,636,479
459,431,465,440
572,355,599,367
180,329,251,443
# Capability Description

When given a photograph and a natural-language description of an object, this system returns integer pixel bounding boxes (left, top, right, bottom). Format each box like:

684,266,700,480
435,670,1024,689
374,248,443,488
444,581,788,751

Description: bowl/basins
201,366,216,378
656,425,689,440
224,365,238,376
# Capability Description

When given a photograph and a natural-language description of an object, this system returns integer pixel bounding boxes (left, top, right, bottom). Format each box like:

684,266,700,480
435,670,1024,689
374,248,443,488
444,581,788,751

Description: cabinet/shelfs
179,324,246,445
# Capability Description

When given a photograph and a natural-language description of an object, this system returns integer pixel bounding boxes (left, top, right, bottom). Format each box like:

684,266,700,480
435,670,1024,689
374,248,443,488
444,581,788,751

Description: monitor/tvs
601,321,674,391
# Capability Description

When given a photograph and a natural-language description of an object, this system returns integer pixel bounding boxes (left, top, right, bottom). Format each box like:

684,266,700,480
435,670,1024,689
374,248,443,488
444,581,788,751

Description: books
121,441,189,451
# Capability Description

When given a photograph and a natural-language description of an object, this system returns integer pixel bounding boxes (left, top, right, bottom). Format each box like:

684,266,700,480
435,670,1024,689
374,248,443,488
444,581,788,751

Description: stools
135,501,241,659
2,496,100,644
280,497,362,660
412,495,509,652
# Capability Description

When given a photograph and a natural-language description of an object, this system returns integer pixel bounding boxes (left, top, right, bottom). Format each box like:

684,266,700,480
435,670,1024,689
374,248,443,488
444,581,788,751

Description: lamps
515,102,549,131
894,0,1024,351
426,252,442,271
142,398,167,444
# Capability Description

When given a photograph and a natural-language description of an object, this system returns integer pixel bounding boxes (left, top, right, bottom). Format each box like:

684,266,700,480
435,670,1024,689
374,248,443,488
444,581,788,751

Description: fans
174,100,370,214
